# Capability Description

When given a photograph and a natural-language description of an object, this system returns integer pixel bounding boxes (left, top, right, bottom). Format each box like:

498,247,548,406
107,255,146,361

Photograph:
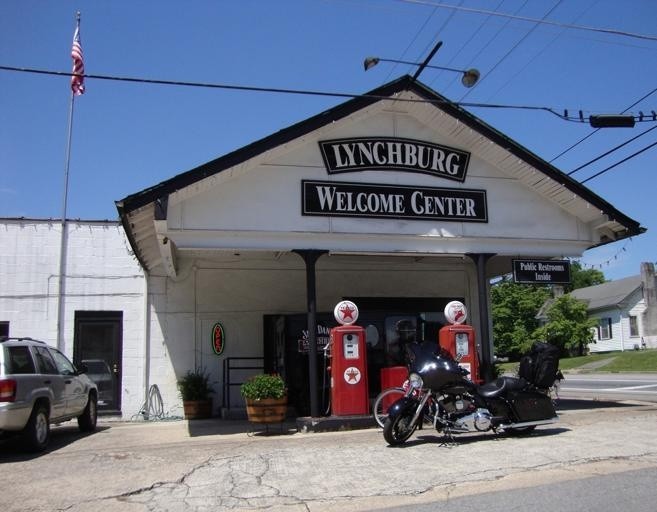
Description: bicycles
373,387,412,431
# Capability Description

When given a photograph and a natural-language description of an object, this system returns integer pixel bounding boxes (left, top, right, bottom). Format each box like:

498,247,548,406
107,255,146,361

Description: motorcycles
382,338,562,446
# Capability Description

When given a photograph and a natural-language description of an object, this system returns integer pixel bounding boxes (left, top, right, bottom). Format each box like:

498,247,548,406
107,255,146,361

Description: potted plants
174,363,222,421
238,369,292,424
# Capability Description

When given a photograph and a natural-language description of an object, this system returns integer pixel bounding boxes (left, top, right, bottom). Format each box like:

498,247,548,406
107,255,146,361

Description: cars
0,336,101,453
81,358,112,403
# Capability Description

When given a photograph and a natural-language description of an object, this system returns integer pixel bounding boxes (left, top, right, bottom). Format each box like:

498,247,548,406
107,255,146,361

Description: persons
641,337,646,351
